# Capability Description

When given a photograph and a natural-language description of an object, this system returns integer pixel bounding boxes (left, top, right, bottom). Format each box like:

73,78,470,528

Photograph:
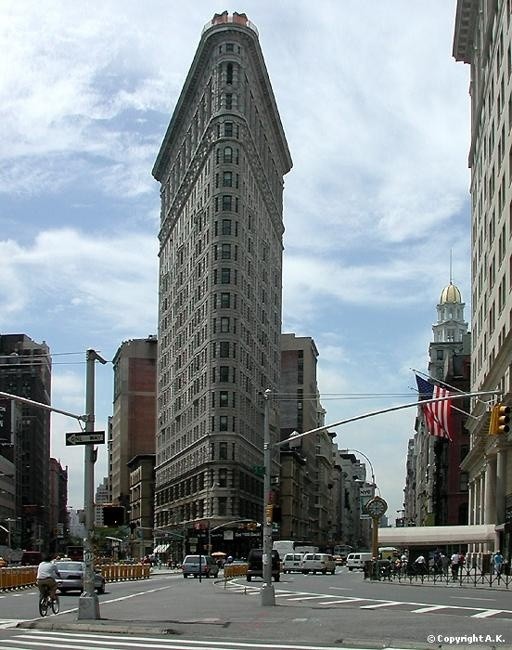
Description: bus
334,544,356,559
64,546,83,561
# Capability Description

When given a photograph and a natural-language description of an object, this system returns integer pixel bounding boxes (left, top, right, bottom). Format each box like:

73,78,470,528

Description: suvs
20,552,42,566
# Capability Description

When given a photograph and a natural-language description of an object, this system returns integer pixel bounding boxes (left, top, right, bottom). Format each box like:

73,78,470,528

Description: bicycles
39,578,63,617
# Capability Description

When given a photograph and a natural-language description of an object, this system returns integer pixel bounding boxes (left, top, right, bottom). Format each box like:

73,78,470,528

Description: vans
346,553,372,571
282,553,337,575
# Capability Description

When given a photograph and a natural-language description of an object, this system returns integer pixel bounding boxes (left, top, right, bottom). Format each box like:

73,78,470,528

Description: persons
93,551,234,570
383,550,504,583
35,553,62,612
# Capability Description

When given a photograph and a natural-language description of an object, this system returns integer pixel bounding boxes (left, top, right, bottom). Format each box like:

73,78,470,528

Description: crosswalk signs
65,432,106,446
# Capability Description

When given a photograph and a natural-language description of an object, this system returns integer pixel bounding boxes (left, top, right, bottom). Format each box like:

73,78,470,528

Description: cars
332,554,343,566
246,549,283,582
35,559,107,593
181,555,219,578
0,557,8,568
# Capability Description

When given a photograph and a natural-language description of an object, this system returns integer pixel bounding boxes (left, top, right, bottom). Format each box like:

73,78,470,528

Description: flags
417,391,448,439
415,374,454,441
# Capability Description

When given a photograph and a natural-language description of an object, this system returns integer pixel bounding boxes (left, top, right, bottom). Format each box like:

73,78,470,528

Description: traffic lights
489,402,511,436
